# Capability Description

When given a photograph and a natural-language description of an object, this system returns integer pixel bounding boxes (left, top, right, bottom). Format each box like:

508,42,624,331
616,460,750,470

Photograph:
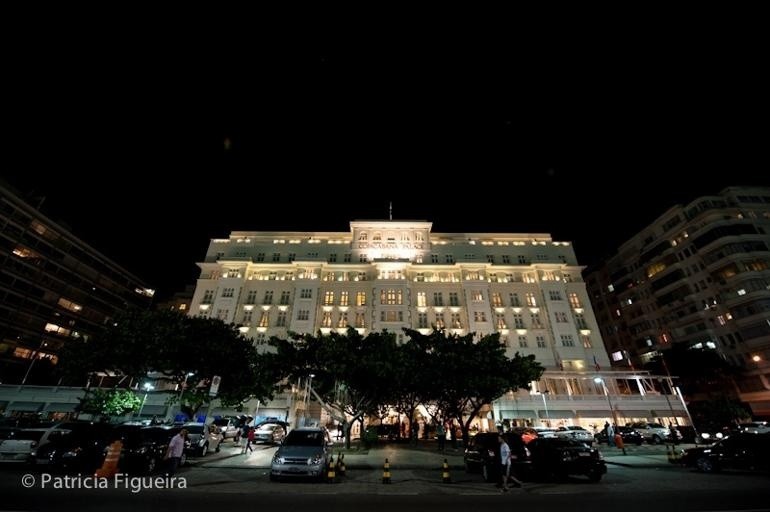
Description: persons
148,414,160,426
165,418,171,425
498,435,523,491
163,427,187,487
241,421,250,454
493,425,506,488
603,420,617,447
392,419,460,453
244,426,256,453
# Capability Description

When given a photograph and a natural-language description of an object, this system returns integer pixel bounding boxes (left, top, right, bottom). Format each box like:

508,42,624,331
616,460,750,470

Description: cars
594,425,644,446
682,422,769,472
0,418,244,477
631,422,683,444
270,427,333,480
463,425,607,484
252,423,285,445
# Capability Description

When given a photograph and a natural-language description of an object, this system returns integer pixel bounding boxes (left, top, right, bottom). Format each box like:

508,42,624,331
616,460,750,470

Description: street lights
536,390,551,428
137,383,155,419
307,374,315,415
594,378,621,434
179,372,194,402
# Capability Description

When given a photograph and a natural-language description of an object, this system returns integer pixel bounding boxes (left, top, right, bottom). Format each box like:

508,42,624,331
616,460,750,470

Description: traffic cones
327,452,346,483
666,444,679,462
442,459,452,483
382,458,391,484
